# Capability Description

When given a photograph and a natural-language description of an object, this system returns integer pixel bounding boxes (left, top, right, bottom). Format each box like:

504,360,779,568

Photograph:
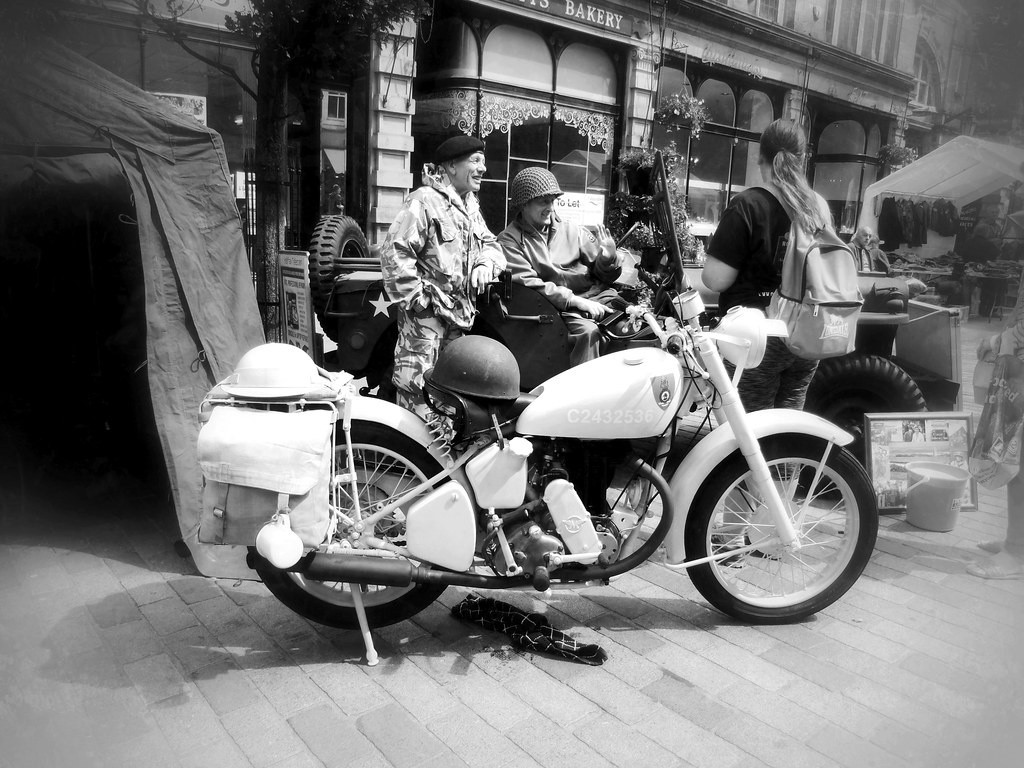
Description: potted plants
608,143,708,273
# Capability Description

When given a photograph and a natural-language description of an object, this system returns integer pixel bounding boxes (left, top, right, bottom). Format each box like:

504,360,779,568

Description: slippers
978,540,1003,553
966,560,1022,580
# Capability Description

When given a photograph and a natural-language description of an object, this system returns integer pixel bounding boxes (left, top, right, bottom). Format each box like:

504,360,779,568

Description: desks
863,268,1021,323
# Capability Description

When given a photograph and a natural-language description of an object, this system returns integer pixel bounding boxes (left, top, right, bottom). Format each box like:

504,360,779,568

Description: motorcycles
196,150,961,665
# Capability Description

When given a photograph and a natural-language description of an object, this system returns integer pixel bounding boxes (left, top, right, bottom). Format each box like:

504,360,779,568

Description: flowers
656,92,714,140
875,144,918,171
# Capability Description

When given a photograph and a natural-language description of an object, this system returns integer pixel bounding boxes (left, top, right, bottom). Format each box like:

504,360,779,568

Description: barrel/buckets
903,461,971,531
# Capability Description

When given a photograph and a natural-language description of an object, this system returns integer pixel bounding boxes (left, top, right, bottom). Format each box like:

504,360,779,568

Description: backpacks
749,180,866,359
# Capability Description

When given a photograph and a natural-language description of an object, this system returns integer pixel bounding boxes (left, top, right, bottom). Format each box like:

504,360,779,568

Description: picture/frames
862,412,979,514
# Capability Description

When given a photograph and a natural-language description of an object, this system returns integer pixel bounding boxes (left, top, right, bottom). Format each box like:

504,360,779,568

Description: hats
433,136,485,164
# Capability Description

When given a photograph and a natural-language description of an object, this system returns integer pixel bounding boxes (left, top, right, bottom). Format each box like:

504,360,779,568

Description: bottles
973,351,996,406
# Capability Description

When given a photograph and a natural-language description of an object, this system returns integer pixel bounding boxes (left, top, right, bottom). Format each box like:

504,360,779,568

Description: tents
860,134,1024,315
0,28,267,580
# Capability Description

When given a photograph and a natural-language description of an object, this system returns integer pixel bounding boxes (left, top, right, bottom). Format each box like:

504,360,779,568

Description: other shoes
712,527,745,567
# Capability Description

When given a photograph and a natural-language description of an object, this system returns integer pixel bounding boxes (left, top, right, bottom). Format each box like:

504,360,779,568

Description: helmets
509,166,564,207
431,334,521,399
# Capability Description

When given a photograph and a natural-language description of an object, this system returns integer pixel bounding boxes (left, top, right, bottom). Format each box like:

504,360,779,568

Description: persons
839,228,890,275
701,120,830,566
974,203,1006,317
383,136,506,451
494,168,623,369
965,216,1024,582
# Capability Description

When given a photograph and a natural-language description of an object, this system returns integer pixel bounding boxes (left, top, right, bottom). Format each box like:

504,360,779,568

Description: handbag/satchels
968,329,1024,491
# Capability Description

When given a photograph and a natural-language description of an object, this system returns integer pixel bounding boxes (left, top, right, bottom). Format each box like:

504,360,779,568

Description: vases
669,114,691,125
888,157,903,165
